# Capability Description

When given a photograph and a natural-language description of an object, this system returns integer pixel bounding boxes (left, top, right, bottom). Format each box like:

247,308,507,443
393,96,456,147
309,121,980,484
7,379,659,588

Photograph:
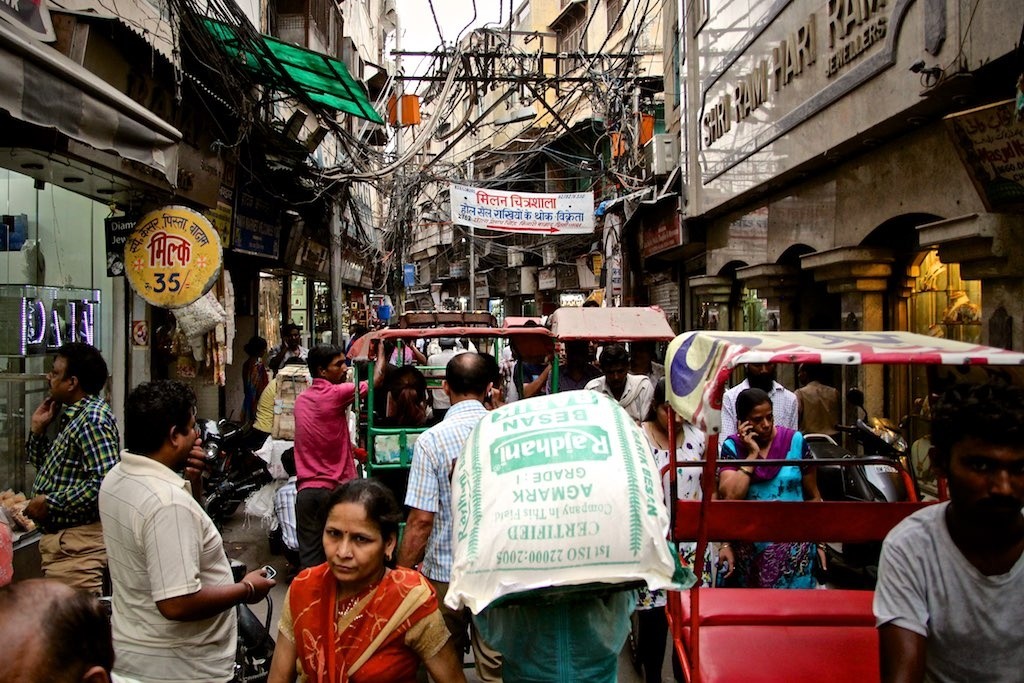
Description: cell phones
262,565,276,580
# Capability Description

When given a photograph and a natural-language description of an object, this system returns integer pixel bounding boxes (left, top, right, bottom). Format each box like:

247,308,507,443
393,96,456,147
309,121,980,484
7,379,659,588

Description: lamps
271,109,309,142
300,124,330,155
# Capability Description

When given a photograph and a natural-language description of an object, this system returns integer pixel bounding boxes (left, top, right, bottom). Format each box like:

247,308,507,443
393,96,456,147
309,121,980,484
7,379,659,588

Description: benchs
672,497,941,683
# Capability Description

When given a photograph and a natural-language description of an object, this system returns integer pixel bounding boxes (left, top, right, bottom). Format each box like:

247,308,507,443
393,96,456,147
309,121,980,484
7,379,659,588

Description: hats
439,338,454,345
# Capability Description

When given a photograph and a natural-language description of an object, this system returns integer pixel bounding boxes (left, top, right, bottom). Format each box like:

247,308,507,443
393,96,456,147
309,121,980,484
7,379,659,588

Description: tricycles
345,324,561,519
632,328,1024,683
545,306,675,400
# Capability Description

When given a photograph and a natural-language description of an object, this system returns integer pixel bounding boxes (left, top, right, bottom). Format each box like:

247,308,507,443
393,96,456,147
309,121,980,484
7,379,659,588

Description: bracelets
739,467,753,478
817,542,826,553
720,544,732,550
242,580,254,604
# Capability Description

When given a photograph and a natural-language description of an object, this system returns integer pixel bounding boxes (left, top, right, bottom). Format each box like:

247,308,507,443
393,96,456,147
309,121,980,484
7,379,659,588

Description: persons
463,394,640,683
635,375,735,683
240,307,940,587
23,343,122,597
267,481,469,683
0,578,116,683
98,382,276,683
719,388,827,591
872,404,1024,683
395,352,510,671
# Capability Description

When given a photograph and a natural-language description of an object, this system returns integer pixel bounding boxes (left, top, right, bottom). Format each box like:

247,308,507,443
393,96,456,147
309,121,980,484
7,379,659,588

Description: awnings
193,13,388,126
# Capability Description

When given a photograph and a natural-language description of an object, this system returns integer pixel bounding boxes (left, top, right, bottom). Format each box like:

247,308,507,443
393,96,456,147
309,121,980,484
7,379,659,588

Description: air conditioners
643,133,678,179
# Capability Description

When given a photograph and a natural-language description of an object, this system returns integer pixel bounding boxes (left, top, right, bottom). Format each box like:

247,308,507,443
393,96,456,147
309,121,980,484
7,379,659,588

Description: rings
730,567,735,570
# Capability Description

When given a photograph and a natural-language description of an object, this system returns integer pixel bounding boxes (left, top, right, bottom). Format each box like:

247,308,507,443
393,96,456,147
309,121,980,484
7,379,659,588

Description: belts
39,517,100,534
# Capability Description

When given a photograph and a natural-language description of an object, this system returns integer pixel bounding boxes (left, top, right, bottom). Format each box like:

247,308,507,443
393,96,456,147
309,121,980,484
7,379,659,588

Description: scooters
800,385,936,582
196,410,269,526
